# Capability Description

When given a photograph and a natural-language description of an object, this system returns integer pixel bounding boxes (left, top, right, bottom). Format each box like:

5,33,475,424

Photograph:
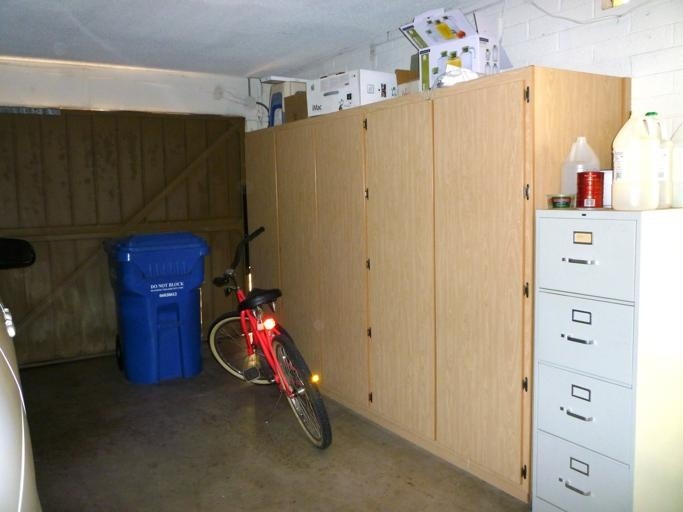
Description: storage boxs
399,8,512,91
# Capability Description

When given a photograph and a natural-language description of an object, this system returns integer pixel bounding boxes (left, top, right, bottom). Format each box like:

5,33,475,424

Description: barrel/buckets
561,136,599,207
612,111,683,209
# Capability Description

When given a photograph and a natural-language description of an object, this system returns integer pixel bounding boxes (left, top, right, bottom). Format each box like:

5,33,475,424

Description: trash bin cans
103,231,211,385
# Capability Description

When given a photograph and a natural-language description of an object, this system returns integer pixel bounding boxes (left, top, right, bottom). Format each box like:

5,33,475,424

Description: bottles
424,16,498,88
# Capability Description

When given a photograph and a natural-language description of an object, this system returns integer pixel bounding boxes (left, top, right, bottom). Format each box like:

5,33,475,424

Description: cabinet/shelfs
244,63,630,503
533,206,683,512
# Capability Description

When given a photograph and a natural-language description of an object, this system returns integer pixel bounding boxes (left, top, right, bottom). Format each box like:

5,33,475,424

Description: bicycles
207,225,333,453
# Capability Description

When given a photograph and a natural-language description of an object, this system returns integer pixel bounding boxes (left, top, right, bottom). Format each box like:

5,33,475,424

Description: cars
0,237,46,511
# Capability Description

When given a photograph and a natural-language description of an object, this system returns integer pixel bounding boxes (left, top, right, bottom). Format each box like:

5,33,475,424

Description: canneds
552,197,572,208
576,172,604,209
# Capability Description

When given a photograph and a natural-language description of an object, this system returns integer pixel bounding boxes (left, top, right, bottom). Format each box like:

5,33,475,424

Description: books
260,76,307,84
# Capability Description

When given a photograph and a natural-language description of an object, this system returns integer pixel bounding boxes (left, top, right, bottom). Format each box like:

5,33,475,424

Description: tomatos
456,31,465,39
